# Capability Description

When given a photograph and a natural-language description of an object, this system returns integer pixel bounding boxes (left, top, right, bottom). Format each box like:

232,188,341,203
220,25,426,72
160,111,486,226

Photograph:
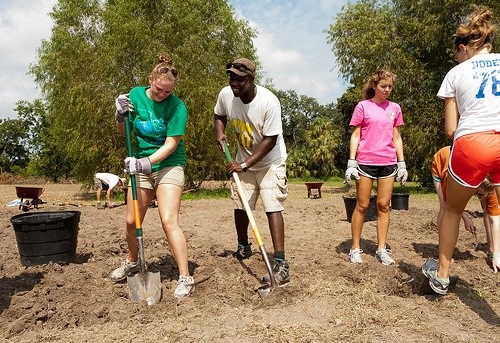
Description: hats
119,177,127,187
225,58,257,78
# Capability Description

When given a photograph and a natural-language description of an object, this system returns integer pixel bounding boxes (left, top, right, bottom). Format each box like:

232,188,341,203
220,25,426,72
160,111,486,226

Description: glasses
225,62,249,71
159,67,178,77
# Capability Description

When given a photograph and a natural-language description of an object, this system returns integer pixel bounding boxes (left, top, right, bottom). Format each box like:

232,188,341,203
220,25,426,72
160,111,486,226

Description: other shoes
97,201,104,208
267,255,290,285
105,202,115,207
231,242,252,260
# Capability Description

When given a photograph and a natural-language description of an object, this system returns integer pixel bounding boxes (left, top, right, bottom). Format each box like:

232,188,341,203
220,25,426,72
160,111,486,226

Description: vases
9,210,81,268
390,193,410,211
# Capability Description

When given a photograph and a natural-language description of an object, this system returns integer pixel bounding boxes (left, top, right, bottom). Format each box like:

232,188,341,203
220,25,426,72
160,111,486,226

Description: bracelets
240,162,247,170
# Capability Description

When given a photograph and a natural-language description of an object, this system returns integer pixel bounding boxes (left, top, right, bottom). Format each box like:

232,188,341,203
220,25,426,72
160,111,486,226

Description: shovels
123,94,161,305
221,139,291,302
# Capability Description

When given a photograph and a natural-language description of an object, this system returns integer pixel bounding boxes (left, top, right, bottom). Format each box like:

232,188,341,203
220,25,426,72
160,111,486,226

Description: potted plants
332,112,380,224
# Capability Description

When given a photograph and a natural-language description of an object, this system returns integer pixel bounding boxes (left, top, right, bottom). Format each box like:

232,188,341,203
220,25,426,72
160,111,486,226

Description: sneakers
110,256,141,283
173,275,195,298
349,248,364,265
374,248,395,265
421,257,450,296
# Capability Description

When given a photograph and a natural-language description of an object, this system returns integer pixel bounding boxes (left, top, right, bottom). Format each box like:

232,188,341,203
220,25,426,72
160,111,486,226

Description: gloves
123,157,153,176
394,161,408,187
113,94,134,122
344,159,361,186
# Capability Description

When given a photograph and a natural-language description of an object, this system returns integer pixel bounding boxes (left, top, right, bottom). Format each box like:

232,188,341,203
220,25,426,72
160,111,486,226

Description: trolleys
16,186,45,211
305,182,324,198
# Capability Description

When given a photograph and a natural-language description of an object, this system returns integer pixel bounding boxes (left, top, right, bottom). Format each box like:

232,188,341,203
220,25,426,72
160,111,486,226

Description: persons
94,172,126,209
343,69,408,265
214,57,291,285
422,4,500,294
110,53,195,299
431,146,500,274
